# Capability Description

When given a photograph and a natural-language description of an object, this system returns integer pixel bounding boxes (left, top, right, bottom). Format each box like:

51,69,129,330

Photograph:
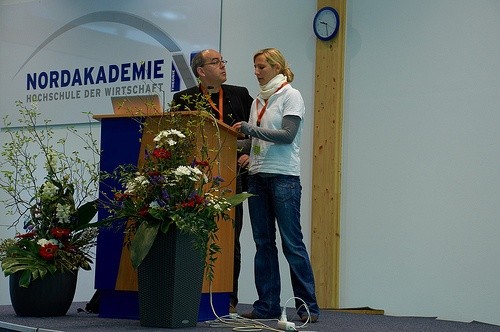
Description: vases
138,222,206,327
9,268,77,317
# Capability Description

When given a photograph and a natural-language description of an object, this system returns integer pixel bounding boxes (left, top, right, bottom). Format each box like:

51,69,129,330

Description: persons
231,48,319,322
168,49,254,313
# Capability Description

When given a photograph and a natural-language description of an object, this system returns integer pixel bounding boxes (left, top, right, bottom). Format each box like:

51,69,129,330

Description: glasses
200,60,227,67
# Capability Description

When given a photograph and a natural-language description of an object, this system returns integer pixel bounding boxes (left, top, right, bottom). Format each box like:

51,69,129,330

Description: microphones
225,96,237,120
204,86,214,97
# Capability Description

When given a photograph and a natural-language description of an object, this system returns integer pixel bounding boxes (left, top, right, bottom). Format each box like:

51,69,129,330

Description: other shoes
229,303,237,313
302,315,319,323
241,311,261,319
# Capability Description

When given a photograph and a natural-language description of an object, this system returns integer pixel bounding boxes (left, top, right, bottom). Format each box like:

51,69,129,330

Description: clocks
313,7,340,41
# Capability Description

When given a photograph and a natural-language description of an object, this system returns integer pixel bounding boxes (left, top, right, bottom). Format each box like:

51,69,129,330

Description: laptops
111,94,162,114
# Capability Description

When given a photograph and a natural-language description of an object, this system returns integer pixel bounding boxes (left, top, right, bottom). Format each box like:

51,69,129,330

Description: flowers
0,99,111,288
87,77,259,280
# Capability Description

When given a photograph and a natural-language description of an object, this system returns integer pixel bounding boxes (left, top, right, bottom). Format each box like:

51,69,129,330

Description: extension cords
278,321,296,330
